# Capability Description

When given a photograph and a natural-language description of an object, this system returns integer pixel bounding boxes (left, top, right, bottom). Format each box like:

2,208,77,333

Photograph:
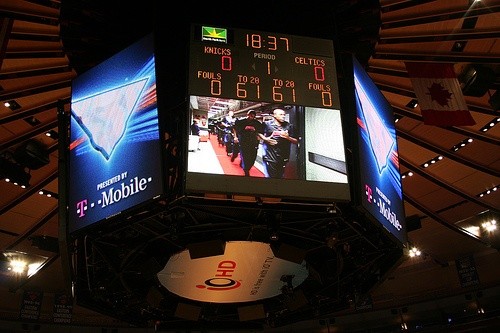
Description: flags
402,62,477,128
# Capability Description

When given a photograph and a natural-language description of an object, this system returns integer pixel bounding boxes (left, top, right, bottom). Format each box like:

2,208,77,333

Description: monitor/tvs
183,22,352,204
67,32,164,235
341,52,411,249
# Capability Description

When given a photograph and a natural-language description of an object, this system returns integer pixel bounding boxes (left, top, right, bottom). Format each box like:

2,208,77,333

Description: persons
258,108,298,178
236,110,263,176
190,119,200,136
207,110,242,167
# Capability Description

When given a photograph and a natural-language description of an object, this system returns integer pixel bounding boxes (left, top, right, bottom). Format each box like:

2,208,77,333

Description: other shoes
245,171,250,176
230,155,236,162
239,161,242,168
